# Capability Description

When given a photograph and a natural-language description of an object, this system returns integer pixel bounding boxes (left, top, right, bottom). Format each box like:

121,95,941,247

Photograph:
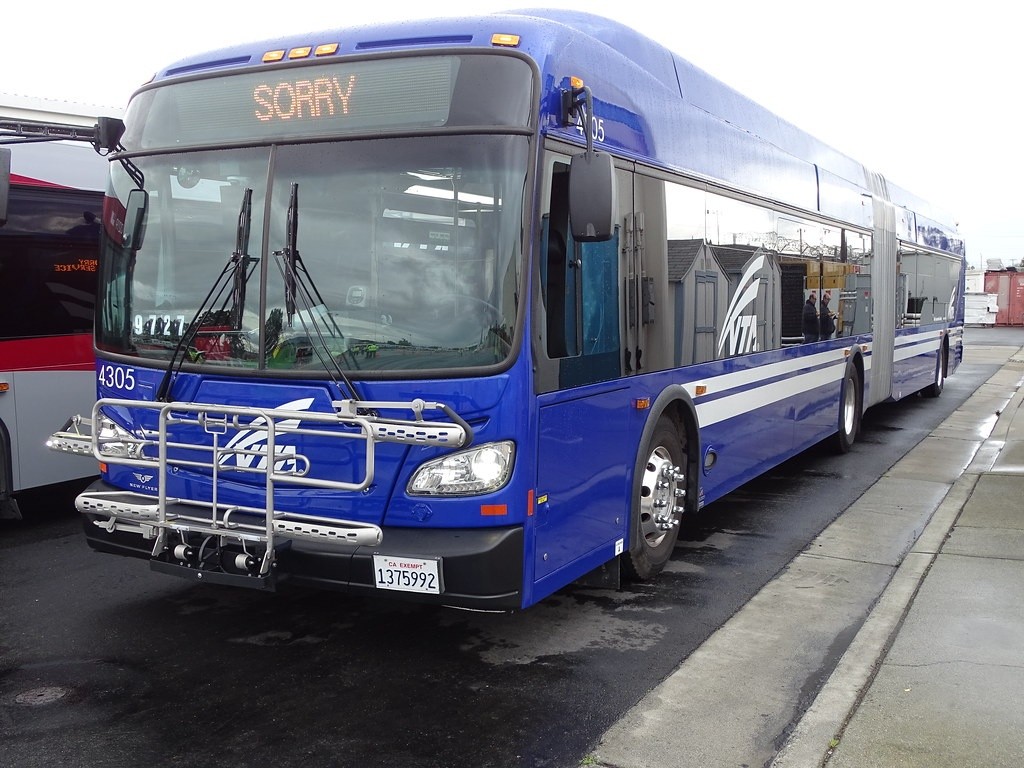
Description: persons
820,293,840,341
802,293,819,345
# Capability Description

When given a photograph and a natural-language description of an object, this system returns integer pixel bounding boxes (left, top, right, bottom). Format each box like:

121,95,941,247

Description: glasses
826,298,831,300
813,298,816,300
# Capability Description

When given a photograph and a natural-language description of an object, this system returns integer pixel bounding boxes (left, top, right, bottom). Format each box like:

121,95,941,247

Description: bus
0,132,486,522
42,4,973,618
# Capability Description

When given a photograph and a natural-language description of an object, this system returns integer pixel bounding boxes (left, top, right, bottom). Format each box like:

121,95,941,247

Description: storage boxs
807,261,855,331
